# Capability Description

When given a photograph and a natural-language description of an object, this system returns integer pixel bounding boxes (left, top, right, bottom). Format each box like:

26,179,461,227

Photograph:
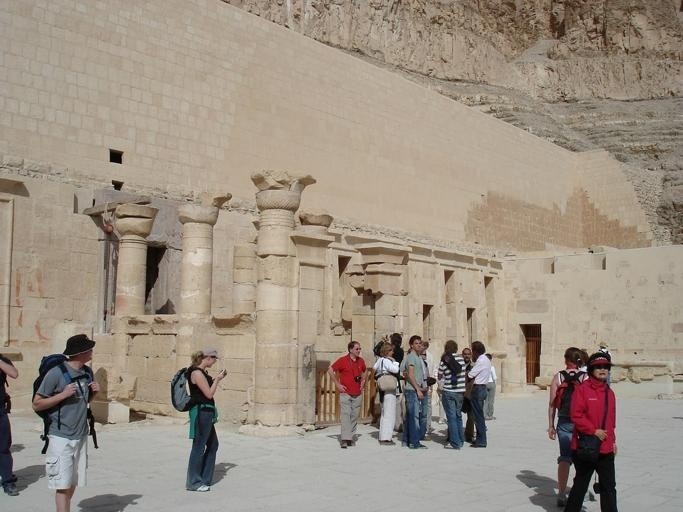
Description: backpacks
33,354,93,425
553,370,588,420
171,367,208,412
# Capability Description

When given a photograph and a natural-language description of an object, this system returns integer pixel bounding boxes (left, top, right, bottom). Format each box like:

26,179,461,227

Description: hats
590,356,610,368
62,334,95,357
201,346,220,359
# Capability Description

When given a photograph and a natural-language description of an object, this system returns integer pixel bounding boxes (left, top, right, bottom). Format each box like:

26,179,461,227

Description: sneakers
379,436,486,449
341,440,348,448
185,484,210,492
3,482,18,496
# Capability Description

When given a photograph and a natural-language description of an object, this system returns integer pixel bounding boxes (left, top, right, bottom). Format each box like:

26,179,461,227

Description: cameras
355,375,360,381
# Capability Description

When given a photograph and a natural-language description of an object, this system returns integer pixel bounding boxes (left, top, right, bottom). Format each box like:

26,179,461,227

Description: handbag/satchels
574,437,600,465
377,375,397,393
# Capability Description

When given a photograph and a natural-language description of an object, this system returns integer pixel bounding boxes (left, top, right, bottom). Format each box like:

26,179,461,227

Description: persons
548,341,616,511
32,334,100,512
326,334,496,448
185,348,226,492
0,352,21,497
